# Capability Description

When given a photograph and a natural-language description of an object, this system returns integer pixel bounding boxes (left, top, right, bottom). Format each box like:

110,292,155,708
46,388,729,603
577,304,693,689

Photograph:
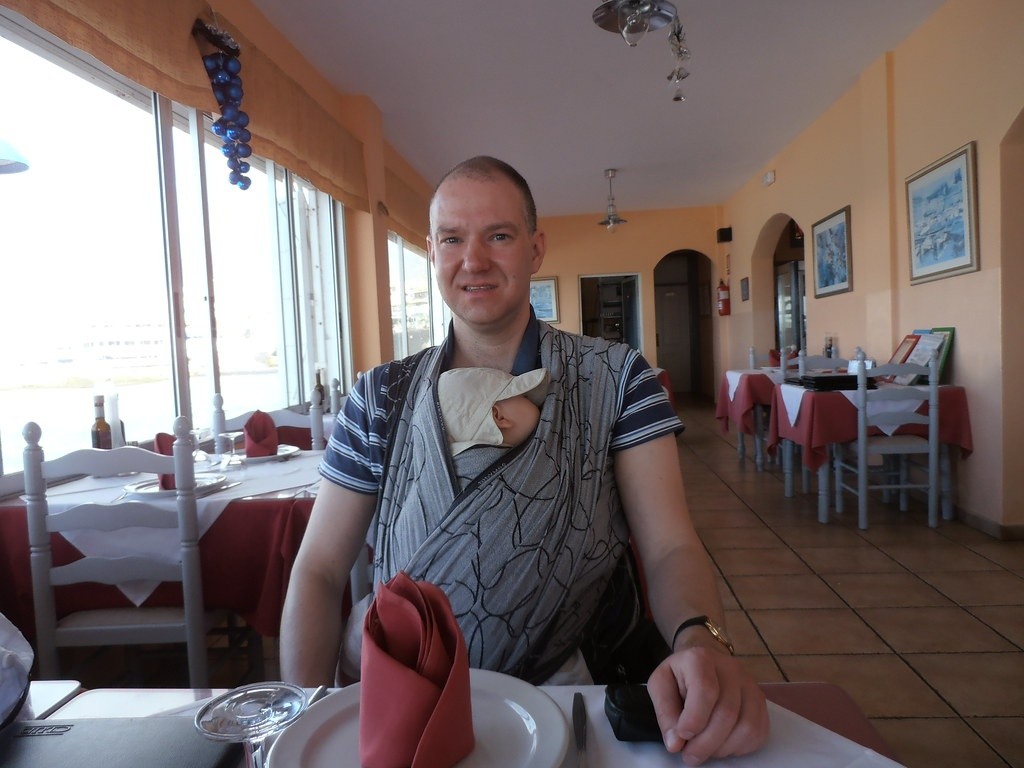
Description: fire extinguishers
717,278,730,316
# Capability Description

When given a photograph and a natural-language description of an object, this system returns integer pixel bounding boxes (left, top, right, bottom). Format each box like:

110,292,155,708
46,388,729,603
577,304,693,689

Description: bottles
91,395,125,454
314,368,325,406
825,336,832,358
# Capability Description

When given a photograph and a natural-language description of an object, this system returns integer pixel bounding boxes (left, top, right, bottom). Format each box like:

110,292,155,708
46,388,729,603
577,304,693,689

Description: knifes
572,693,587,768
195,481,242,499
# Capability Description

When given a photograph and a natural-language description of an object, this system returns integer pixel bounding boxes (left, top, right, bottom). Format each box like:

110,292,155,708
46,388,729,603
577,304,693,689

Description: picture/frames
811,205,851,298
529,277,560,324
877,327,955,384
904,143,979,284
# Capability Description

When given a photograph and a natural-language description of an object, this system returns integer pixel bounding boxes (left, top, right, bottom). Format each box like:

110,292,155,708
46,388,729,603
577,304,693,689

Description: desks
715,367,851,477
769,384,971,526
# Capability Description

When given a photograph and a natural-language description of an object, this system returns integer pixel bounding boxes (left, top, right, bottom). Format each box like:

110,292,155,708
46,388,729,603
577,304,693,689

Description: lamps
599,168,628,232
593,0,691,102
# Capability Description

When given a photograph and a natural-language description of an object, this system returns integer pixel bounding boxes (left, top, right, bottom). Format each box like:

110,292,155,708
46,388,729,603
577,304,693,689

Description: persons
279,155,769,768
436,367,551,492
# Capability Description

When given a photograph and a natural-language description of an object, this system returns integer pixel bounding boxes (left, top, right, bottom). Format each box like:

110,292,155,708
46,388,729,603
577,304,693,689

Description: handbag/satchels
580,547,672,687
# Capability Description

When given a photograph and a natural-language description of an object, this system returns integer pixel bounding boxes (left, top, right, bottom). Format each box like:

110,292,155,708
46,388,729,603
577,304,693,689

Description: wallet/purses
605,684,664,742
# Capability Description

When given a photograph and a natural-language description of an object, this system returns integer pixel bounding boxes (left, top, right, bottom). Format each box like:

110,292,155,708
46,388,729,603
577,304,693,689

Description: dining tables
0,681,902,766
279,411,341,451
0,449,326,690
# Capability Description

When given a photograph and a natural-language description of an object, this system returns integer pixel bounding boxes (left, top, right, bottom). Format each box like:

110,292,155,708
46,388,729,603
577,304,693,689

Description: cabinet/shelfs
597,278,626,342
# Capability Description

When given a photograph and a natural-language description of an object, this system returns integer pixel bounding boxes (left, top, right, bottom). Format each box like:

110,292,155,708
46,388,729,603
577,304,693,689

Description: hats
436,367,546,456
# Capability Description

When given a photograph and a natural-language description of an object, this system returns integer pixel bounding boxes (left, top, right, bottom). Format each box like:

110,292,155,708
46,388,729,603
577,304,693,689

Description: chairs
19,414,213,683
748,346,938,529
211,394,324,455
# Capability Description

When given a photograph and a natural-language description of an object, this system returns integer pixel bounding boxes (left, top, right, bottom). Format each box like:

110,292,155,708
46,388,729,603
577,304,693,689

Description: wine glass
218,431,244,472
189,427,211,465
194,681,309,768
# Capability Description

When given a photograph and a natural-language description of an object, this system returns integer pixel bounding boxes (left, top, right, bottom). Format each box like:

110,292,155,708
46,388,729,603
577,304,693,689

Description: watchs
672,615,735,656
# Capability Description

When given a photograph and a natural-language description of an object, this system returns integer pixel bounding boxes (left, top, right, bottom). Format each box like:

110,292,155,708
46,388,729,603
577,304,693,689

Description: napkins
154,432,176,494
351,573,474,765
244,410,279,458
787,350,797,368
769,349,781,369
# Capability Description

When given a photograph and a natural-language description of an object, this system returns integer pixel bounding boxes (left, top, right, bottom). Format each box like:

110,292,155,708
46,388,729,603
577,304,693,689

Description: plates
236,444,300,464
123,473,226,498
266,668,570,768
0,671,31,730
